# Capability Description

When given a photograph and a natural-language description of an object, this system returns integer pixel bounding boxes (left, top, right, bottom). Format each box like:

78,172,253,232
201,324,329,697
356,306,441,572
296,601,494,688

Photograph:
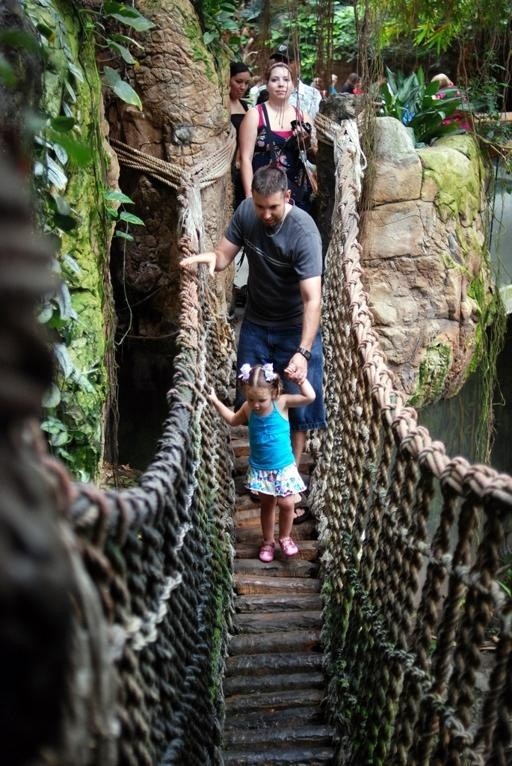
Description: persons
227,50,472,207
205,364,318,563
178,164,325,472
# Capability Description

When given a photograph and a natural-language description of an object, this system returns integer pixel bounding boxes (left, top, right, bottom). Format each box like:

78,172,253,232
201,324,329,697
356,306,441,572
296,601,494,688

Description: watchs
297,348,312,360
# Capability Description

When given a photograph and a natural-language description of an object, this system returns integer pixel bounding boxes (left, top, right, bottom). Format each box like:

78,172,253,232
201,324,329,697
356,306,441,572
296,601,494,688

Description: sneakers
259,539,275,563
278,536,298,556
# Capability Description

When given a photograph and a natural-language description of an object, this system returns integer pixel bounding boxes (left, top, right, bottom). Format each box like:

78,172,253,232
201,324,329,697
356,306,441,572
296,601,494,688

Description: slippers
249,489,311,524
